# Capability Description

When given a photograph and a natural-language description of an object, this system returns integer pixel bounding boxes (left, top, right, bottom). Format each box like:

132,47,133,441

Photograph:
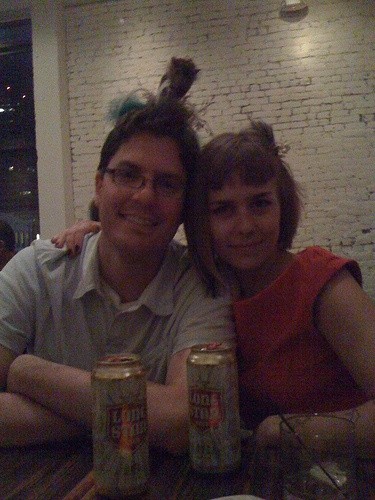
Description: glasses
106,168,183,197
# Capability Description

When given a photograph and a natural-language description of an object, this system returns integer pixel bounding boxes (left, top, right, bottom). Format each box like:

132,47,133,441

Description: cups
278,413,356,500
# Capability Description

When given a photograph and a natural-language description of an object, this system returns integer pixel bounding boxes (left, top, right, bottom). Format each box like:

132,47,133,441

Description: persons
0,97,240,458
0,217,17,271
49,123,375,457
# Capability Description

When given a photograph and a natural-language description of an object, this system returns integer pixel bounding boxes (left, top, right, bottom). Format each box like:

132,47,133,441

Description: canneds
186,341,240,475
89,352,150,496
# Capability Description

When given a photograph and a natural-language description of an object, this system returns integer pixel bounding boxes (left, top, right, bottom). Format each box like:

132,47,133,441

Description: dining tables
0,430,375,500
